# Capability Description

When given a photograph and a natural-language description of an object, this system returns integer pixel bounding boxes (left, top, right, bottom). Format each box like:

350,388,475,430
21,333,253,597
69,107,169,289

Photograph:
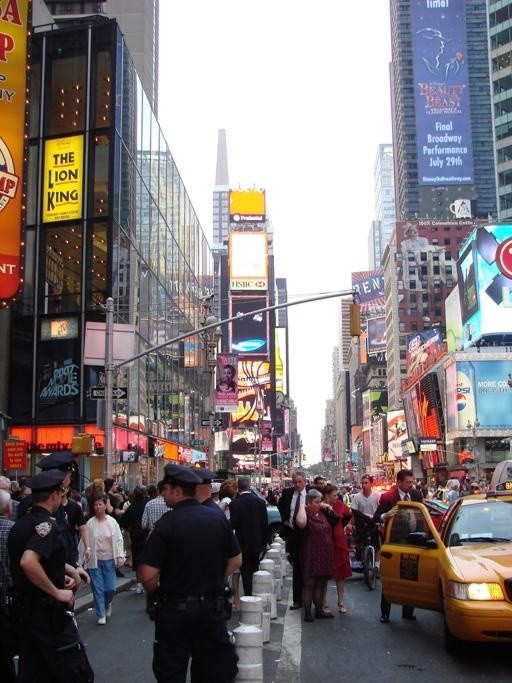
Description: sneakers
106,603,112,617
97,615,106,625
130,583,144,594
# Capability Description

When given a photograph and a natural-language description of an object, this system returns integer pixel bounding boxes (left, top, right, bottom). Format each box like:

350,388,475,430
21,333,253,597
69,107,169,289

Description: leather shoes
289,604,416,622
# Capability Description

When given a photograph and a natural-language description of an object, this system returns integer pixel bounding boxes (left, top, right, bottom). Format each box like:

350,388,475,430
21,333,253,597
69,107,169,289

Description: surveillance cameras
199,294,215,301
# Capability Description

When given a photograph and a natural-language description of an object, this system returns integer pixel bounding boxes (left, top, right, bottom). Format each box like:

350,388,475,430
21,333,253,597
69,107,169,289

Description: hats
26,451,216,494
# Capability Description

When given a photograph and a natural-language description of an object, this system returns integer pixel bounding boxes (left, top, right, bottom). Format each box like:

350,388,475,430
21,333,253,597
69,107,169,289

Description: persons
217,363,236,394
1,449,491,625
140,464,239,681
399,223,429,254
6,467,95,682
262,427,271,442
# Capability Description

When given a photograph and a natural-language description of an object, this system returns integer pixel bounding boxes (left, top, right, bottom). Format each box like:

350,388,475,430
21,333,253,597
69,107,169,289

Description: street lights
104,286,361,474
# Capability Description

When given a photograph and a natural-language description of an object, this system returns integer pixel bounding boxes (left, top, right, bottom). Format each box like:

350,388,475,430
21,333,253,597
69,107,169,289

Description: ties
292,493,301,529
404,495,410,522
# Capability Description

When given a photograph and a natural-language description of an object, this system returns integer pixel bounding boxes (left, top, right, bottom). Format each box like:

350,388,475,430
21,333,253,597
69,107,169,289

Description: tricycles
356,544,378,588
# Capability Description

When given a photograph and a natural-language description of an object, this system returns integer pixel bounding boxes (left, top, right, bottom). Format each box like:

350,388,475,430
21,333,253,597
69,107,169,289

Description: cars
379,461,511,639
211,480,282,544
380,499,446,532
